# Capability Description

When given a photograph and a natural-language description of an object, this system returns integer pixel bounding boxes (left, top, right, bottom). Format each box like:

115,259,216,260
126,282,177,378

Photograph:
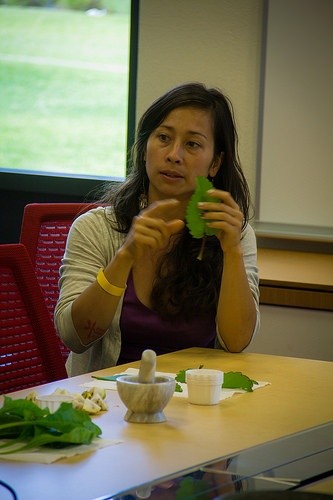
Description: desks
0,347,333,500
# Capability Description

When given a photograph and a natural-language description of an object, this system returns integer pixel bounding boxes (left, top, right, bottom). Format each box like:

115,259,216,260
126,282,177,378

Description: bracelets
96,267,126,297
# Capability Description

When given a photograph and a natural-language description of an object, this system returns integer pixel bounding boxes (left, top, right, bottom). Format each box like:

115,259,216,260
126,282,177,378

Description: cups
36,395,74,413
185,370,224,405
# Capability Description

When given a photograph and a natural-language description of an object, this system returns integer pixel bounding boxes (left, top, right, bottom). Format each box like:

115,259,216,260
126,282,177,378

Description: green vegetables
92,375,129,381
185,176,223,260
175,367,257,392
0,394,102,455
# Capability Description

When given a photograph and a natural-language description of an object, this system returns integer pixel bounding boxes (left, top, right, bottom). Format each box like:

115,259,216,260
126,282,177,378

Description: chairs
21,203,113,363
0,244,68,396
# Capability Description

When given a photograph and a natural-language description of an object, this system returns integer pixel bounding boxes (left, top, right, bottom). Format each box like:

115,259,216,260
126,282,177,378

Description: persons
52,81,261,378
